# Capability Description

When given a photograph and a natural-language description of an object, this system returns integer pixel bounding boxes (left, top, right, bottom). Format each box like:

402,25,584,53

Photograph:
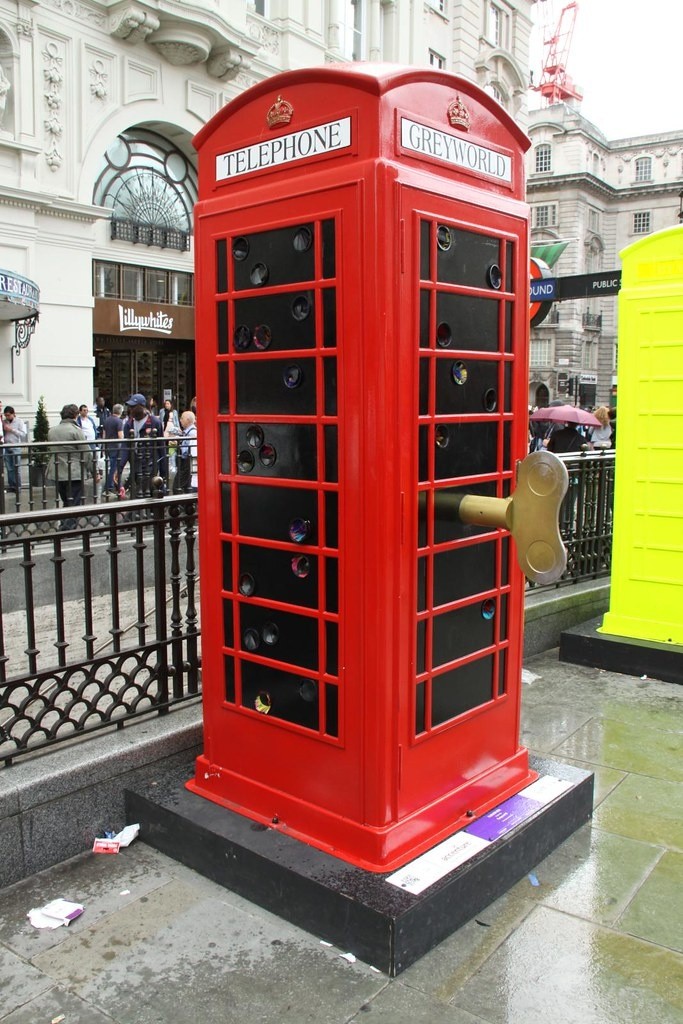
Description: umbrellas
529,403,603,429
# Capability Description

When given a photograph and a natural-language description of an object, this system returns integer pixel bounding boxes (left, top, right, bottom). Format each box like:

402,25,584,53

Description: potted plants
30,396,49,487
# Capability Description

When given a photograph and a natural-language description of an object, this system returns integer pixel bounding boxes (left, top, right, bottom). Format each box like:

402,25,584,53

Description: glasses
82,409,88,412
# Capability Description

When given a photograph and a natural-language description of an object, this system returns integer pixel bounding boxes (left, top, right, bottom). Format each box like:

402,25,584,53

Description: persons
0,394,197,542
529,403,617,537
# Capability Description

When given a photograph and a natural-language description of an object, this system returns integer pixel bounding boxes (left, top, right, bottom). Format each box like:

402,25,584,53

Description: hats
126,394,147,406
